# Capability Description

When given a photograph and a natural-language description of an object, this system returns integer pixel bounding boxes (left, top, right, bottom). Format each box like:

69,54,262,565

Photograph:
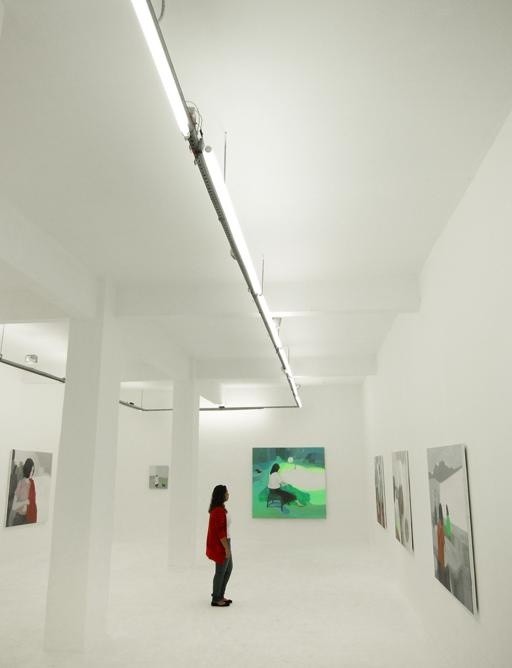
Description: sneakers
211,601,229,606
222,598,232,603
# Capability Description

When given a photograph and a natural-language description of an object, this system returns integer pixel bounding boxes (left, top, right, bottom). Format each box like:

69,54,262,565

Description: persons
11,458,38,525
267,463,304,509
205,485,234,607
